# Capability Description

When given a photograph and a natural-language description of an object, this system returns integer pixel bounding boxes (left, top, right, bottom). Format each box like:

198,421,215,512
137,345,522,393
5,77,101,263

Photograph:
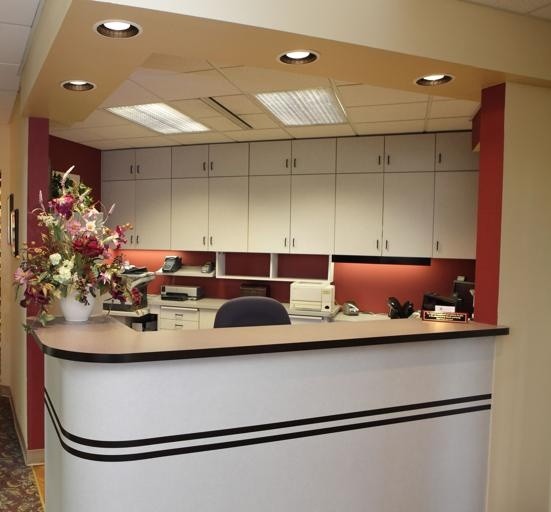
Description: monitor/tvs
453,280,474,314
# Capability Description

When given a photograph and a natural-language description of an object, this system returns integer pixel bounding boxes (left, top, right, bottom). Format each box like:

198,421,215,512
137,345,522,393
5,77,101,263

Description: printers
160,284,202,301
102,264,157,312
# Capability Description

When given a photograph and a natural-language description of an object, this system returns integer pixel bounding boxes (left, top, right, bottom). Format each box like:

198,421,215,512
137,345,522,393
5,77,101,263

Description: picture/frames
6,193,19,256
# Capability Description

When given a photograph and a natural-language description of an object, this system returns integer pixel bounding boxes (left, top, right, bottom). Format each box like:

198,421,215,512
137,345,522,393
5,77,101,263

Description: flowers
12,161,143,335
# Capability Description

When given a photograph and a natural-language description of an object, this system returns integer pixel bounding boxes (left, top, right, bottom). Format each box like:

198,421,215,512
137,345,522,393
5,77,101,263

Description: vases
60,281,95,322
